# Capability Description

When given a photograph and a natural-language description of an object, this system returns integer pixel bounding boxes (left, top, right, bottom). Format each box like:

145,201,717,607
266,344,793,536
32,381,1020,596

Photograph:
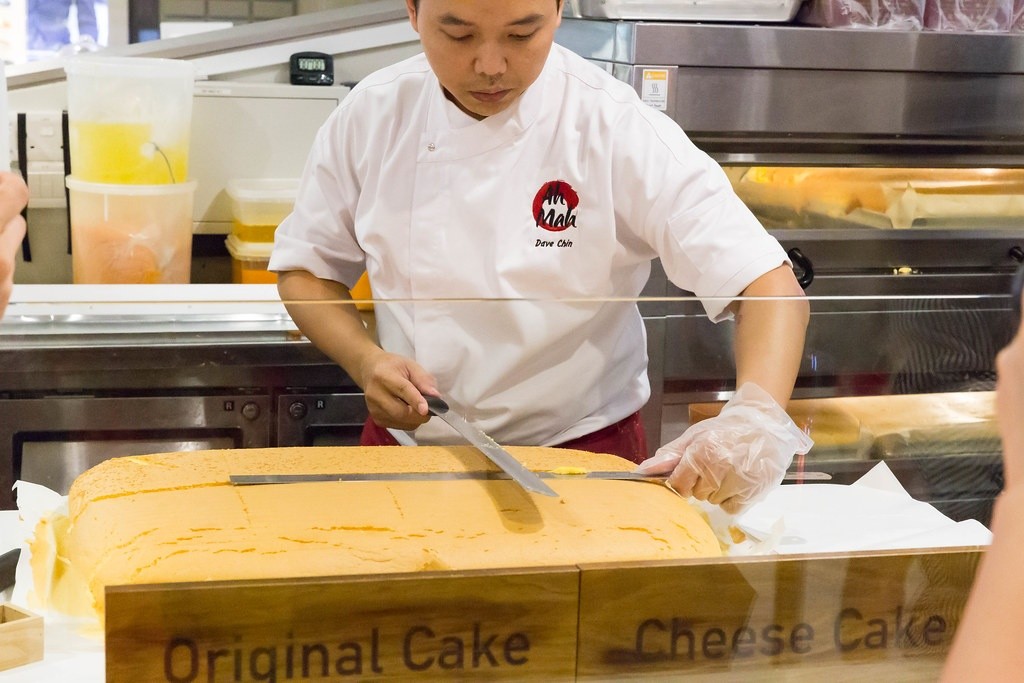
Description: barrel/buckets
65,173,194,286
62,52,197,186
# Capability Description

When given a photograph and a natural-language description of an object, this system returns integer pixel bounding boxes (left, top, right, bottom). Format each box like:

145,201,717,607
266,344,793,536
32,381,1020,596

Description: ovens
553,18,1024,529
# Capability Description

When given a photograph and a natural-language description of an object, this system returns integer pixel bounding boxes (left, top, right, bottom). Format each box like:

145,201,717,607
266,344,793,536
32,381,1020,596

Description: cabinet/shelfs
0,285,373,509
552,17,1023,529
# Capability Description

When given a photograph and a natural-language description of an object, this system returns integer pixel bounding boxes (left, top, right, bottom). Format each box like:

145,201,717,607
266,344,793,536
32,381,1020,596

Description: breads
689,387,1002,452
740,166,1024,219
26,445,726,626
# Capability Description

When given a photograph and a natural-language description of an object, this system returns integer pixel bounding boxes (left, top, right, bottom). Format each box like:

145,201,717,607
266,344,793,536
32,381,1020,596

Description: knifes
418,393,560,500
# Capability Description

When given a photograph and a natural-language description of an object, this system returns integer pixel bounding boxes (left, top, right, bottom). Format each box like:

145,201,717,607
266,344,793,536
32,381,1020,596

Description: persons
270,0,809,515
936,319,1024,681
